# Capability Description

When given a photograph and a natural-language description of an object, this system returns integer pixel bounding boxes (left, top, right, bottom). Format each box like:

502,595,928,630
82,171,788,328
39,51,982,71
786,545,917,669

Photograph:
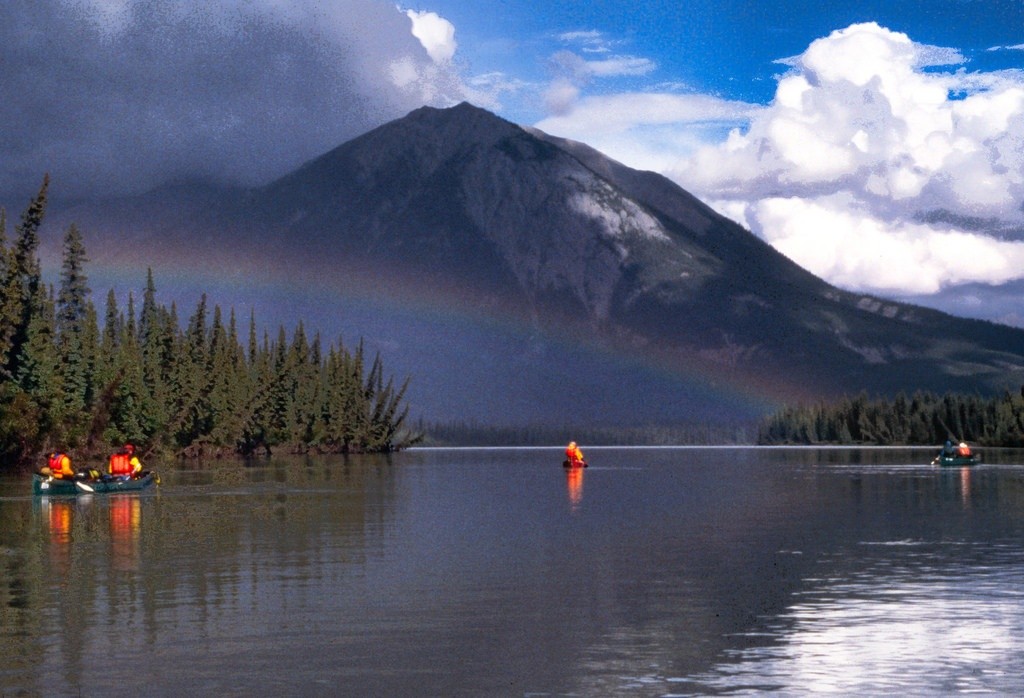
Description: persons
940,441,973,460
49,449,75,481
109,444,143,481
565,441,583,462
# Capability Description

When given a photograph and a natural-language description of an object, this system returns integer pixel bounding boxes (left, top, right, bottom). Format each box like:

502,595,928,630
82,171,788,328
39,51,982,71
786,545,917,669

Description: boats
563,456,588,467
92,470,156,493
30,472,93,495
934,447,983,465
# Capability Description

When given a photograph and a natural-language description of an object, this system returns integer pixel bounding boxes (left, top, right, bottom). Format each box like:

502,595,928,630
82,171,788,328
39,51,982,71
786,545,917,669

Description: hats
54,446,69,455
124,443,135,453
